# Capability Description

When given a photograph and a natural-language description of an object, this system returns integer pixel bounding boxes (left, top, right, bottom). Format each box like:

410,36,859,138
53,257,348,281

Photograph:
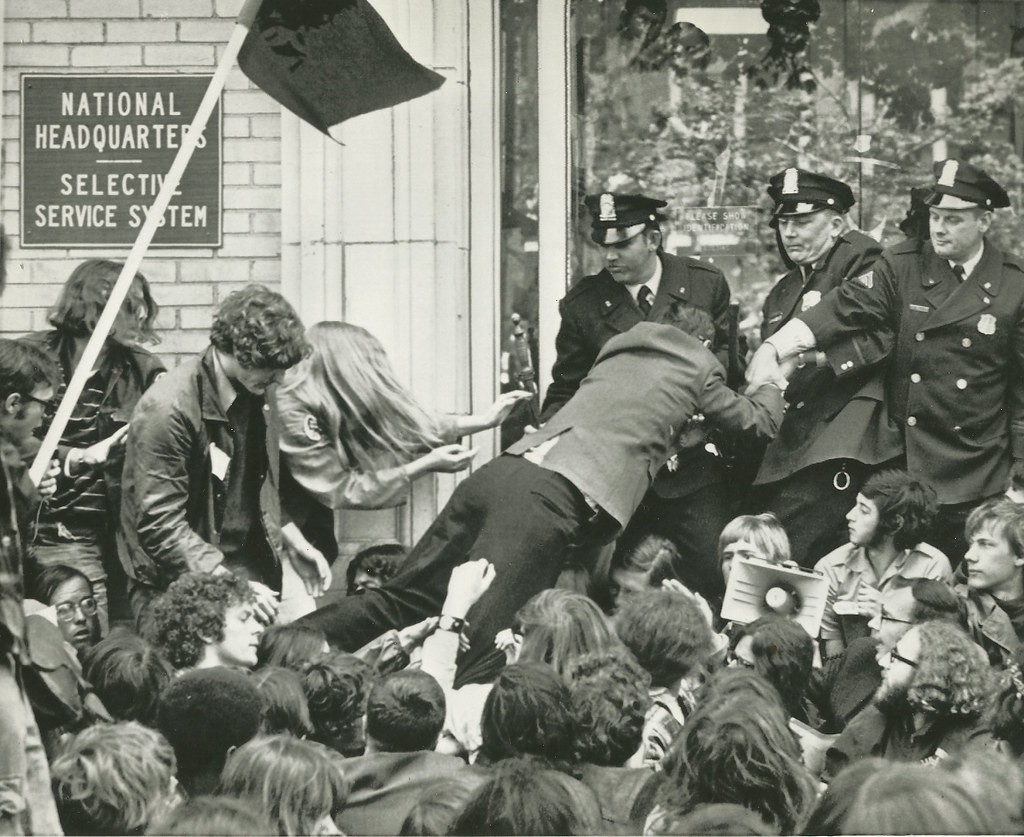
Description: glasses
15,390,55,415
512,623,526,644
870,607,917,625
726,649,755,670
52,596,99,623
890,648,919,668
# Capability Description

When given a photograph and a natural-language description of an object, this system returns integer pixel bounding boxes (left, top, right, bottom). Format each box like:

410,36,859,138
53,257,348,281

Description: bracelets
797,352,806,369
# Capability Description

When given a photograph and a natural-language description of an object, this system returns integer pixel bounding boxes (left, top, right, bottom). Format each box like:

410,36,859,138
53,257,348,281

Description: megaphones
720,557,830,640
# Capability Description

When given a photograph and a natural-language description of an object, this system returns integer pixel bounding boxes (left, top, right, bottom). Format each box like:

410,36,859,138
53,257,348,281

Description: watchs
436,613,465,633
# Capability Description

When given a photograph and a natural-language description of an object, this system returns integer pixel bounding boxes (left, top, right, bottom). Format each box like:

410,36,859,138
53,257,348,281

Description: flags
236,0,447,144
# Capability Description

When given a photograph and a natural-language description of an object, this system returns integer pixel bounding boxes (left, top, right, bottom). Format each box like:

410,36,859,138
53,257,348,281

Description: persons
0,133,1024,835
577,0,824,206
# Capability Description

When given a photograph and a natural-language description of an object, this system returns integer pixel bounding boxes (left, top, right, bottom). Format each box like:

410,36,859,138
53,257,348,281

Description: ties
638,287,652,316
804,264,813,281
952,265,965,285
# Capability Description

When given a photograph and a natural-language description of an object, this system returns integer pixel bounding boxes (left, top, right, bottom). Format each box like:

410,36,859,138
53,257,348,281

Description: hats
769,167,855,216
923,158,1010,213
586,194,667,246
